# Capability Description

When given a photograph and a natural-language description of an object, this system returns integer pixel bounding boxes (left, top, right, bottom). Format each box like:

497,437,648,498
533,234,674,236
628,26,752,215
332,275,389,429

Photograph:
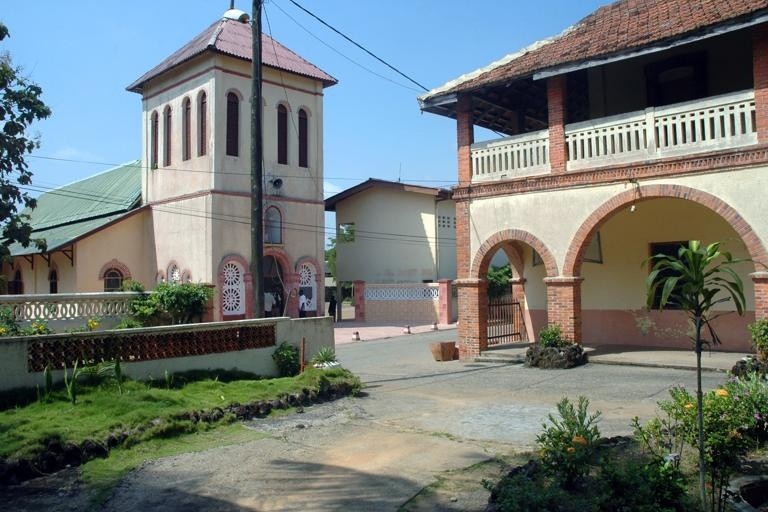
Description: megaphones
272,176,284,189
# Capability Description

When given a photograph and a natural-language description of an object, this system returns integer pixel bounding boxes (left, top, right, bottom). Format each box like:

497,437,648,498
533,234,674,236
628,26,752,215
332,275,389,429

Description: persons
299,289,305,318
327,296,337,322
264,288,282,317
